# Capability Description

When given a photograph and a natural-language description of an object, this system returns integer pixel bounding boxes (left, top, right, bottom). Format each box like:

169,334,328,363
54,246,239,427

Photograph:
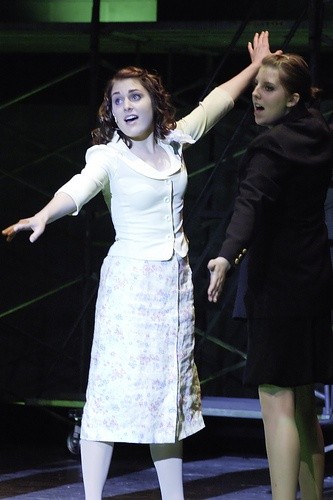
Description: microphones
113,113,117,123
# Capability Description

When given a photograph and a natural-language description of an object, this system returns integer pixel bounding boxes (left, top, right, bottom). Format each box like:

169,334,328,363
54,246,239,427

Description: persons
208,55,333,500
2,30,283,500
